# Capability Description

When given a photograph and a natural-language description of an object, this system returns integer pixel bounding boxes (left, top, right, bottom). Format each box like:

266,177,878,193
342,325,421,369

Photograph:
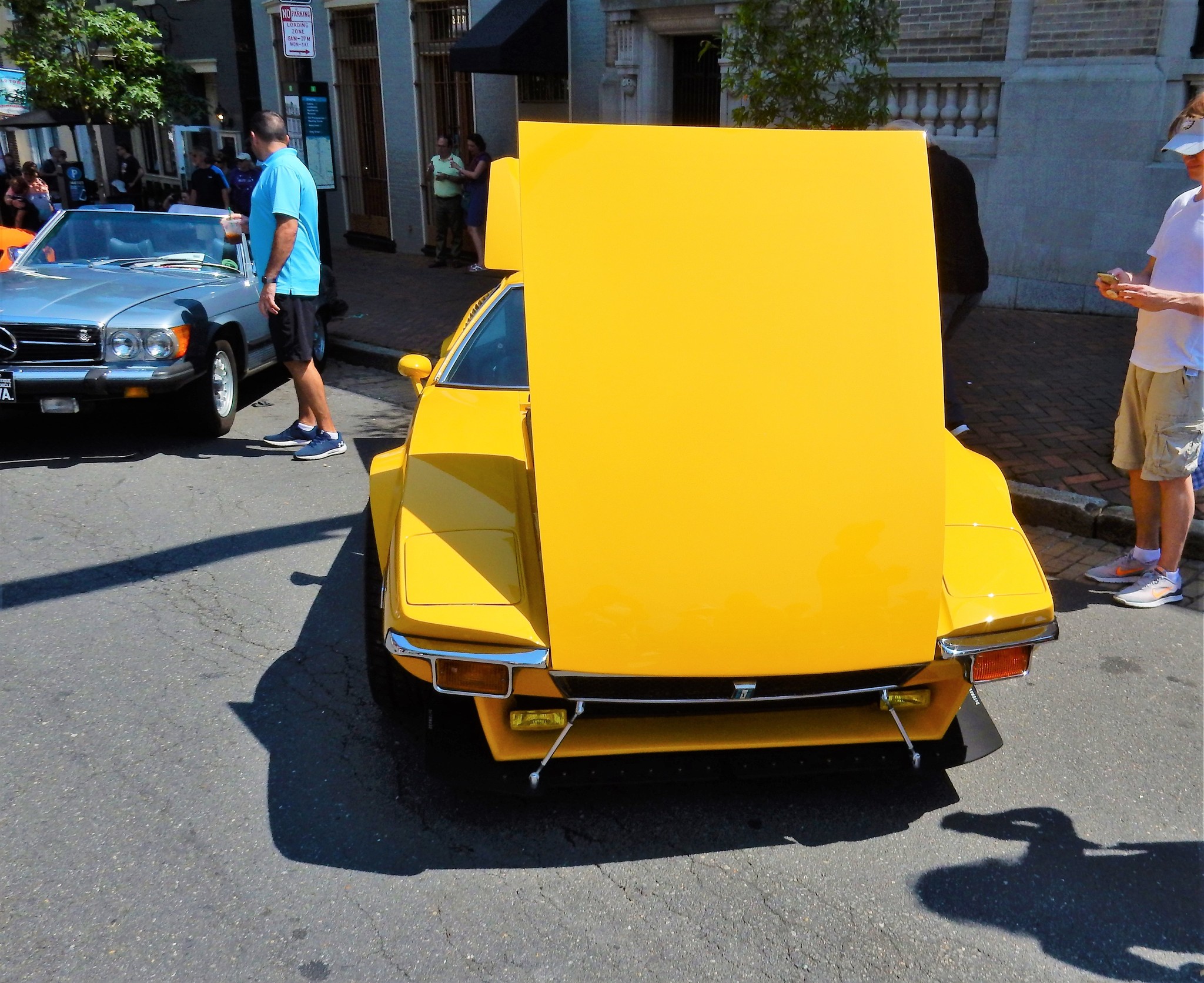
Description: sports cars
362,120,1063,790
0,203,339,442
0,224,56,273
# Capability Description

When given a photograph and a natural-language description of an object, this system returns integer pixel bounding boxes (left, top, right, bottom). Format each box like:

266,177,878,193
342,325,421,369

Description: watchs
261,276,276,284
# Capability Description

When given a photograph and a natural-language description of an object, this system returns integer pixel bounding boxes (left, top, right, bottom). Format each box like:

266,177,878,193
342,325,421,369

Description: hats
22,161,39,174
1161,113,1204,155
237,153,252,160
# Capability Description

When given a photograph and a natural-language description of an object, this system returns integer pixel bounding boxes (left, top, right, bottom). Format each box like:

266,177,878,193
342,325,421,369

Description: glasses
116,148,124,151
214,160,222,164
192,154,199,158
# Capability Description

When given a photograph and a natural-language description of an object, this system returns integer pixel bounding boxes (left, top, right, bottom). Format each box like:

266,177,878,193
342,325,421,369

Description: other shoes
451,257,463,268
469,263,487,272
429,257,445,268
946,420,970,440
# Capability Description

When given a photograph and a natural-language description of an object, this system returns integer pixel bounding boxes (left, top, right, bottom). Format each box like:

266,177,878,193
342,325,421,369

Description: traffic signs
280,3,317,59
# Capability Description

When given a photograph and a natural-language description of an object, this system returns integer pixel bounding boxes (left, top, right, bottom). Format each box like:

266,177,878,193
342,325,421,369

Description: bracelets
460,168,463,172
447,175,450,178
11,200,13,205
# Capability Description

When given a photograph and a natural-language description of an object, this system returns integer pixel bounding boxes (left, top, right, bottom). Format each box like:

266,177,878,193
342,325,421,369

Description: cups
220,219,243,245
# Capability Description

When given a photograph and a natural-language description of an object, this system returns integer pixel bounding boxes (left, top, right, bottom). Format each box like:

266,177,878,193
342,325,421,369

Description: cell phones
1096,273,1119,285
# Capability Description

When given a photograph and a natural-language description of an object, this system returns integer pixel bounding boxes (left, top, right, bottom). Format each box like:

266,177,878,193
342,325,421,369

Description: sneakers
263,420,318,446
1085,548,1160,584
293,429,347,460
1112,567,1183,607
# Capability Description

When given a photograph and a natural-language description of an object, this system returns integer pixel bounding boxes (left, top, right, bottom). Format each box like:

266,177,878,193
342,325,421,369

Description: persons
425,132,493,274
1085,90,1204,608
0,153,54,233
221,109,348,461
116,141,145,211
163,143,262,240
878,119,988,438
38,146,67,204
